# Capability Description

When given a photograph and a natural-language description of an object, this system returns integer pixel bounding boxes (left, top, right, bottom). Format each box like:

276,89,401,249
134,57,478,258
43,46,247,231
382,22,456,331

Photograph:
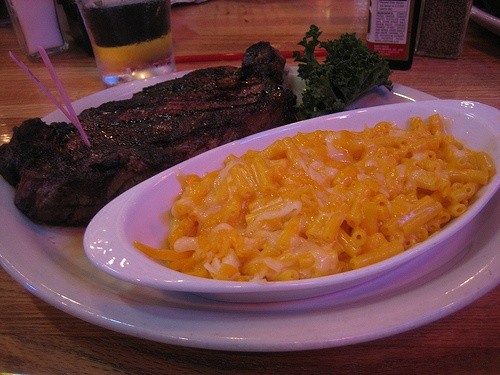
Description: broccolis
291,25,395,117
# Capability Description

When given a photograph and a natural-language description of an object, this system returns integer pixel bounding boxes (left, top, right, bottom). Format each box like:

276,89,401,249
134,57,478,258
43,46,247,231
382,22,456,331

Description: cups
75,0,176,84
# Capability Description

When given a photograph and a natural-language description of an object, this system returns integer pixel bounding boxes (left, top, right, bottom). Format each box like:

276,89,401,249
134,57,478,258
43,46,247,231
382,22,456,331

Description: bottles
414,0,473,60
4,0,71,61
366,0,421,71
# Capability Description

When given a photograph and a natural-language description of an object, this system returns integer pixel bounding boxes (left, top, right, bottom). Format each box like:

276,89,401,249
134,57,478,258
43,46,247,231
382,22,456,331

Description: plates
0,70,500,353
85,99,500,303
